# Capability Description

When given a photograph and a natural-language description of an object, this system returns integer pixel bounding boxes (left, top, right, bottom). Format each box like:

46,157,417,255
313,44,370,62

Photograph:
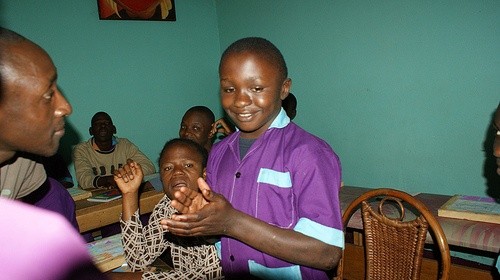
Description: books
86,191,123,202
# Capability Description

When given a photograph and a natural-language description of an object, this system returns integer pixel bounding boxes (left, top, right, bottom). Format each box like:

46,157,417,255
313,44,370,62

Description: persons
161,36,345,279
0,29,297,280
493,107,500,274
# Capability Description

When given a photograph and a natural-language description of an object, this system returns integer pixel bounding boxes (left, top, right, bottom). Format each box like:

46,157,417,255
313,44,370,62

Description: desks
66,173,165,241
339,186,500,280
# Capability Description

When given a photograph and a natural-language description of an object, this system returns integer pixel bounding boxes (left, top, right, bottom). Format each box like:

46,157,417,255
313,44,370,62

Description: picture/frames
97,0,176,21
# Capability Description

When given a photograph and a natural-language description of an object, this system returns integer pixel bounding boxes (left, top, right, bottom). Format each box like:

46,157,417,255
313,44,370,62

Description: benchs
87,233,126,272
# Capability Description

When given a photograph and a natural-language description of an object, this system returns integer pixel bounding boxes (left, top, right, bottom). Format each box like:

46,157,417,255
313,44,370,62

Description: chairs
336,189,451,280
